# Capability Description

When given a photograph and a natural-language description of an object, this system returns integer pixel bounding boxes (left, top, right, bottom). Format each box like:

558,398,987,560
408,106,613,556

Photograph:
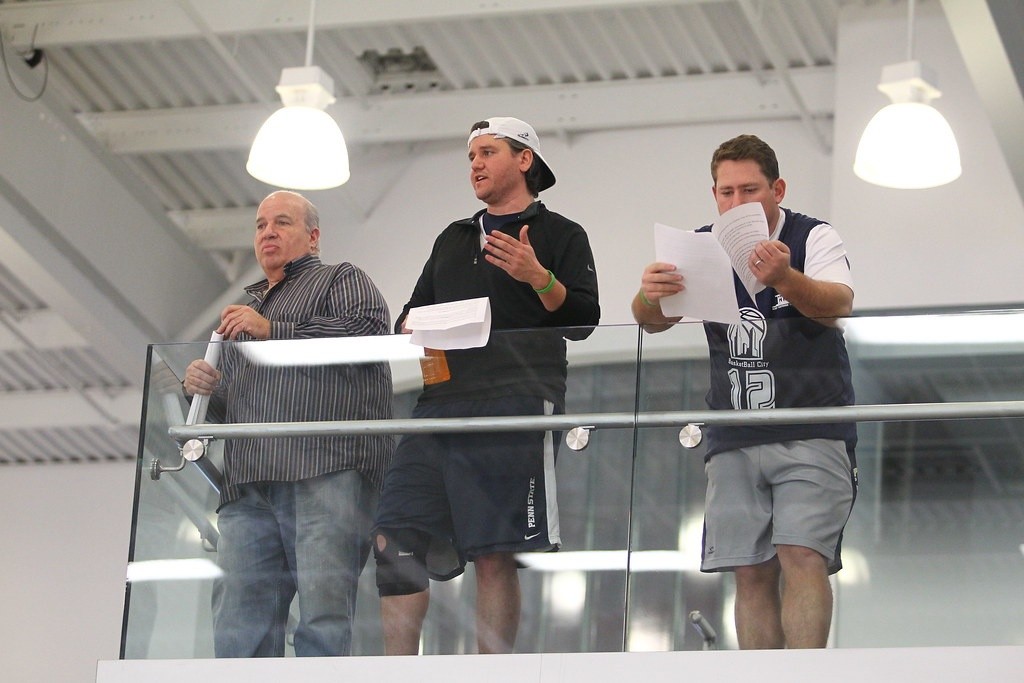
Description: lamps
244,0,353,191
852,0,965,191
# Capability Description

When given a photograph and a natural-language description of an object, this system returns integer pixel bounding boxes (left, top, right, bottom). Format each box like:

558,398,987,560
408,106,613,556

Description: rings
755,260,760,265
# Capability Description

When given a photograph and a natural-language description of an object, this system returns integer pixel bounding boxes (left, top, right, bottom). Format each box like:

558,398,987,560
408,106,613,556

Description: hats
467,117,556,192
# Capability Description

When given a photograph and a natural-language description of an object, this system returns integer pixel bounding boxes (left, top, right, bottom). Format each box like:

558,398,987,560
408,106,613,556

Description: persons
633,135,858,649
373,117,601,656
182,190,393,658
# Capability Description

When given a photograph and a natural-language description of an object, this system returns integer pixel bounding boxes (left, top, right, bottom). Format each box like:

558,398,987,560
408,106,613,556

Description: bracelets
533,270,555,293
639,290,651,305
